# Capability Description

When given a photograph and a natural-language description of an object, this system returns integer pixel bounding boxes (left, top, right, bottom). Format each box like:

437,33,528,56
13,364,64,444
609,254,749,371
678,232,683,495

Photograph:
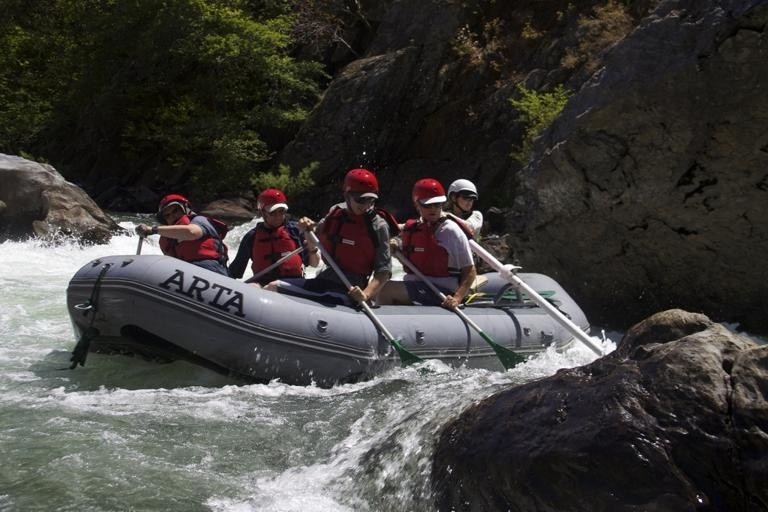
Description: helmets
412,178,447,204
257,189,288,212
342,169,378,199
448,179,478,200
158,194,191,213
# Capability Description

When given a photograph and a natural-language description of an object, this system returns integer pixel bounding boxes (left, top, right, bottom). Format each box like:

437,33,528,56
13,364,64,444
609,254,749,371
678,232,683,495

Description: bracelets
152,224,158,234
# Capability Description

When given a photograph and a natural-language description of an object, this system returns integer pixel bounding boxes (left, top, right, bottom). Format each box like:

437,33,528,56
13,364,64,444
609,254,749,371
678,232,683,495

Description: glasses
162,208,177,220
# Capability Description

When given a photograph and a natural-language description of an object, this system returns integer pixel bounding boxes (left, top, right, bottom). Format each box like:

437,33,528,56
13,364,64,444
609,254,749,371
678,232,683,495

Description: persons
228,168,484,309
135,193,228,277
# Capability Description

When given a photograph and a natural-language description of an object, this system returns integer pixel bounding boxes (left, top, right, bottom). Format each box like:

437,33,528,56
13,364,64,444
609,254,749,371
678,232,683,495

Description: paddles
304,225,423,369
393,247,527,370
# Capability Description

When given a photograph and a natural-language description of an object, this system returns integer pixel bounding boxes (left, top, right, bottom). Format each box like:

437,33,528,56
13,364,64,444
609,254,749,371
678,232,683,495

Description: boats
64,245,594,392
